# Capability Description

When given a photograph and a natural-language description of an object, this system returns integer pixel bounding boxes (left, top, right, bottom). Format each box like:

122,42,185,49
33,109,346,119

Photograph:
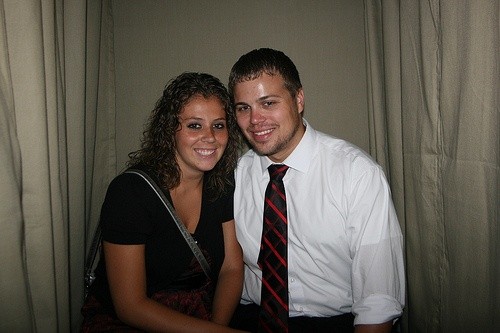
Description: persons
79,71,242,332
226,48,406,332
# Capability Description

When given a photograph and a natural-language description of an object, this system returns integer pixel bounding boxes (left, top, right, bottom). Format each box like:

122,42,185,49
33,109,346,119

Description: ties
257,164,291,333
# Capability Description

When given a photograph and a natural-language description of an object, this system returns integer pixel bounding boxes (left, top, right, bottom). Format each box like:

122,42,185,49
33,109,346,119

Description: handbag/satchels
80,278,218,333
